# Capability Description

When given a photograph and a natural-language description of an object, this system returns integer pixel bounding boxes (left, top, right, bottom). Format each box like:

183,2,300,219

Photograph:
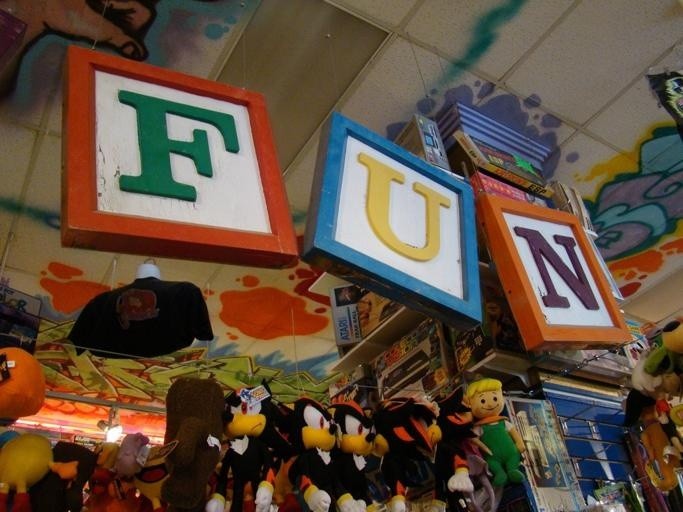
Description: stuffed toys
1,346,526,511
0,345,526,512
622,321,683,491
